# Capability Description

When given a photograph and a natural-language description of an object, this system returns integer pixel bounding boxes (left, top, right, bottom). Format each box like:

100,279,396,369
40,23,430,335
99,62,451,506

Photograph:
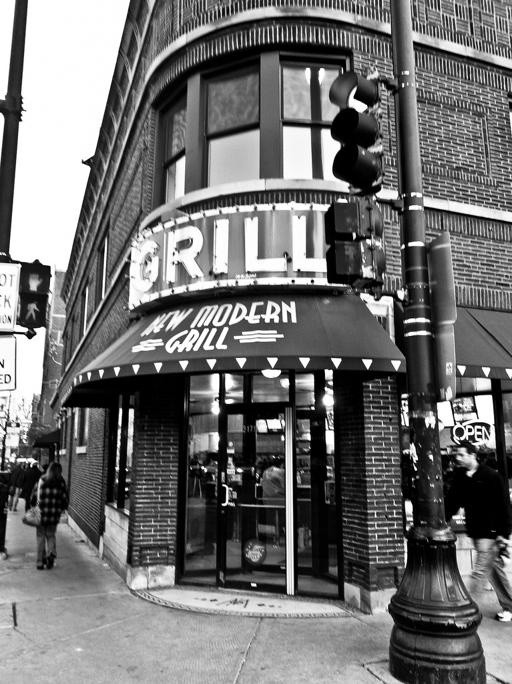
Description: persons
183,440,346,541
398,440,512,540
443,438,512,623
5,454,51,513
29,461,69,568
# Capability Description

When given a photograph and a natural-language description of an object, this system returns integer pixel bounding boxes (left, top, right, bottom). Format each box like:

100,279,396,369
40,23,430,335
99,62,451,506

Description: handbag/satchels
23,506,42,526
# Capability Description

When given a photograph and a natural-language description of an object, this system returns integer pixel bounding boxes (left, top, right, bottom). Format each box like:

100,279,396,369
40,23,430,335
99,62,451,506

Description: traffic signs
0,336,16,391
0,261,20,334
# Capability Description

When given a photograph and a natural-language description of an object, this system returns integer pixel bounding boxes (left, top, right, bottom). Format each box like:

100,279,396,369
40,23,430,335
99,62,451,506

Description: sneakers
496,610,512,622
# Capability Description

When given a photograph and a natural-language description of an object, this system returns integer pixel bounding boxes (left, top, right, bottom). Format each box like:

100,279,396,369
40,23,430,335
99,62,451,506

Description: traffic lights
324,199,386,288
18,259,52,329
329,72,385,197
